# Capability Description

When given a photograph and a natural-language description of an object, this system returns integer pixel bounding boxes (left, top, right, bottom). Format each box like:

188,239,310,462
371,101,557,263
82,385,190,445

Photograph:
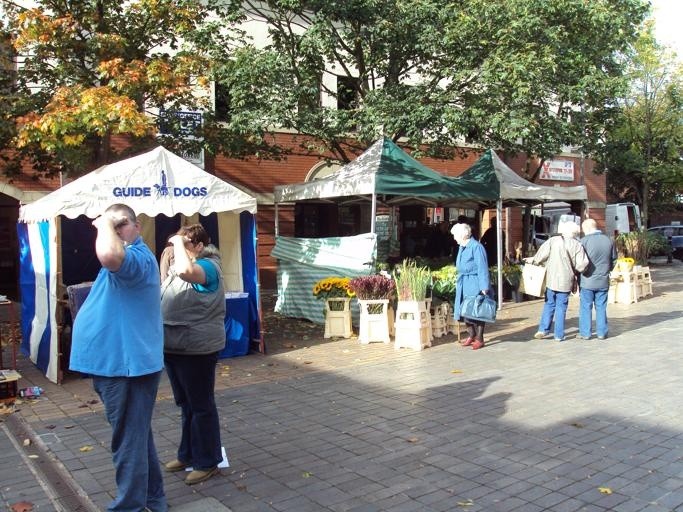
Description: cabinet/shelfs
324,297,353,339
430,305,447,338
447,303,468,337
357,298,390,345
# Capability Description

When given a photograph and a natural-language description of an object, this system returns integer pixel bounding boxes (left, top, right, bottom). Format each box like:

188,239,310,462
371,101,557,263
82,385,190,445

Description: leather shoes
462,336,475,345
472,339,485,350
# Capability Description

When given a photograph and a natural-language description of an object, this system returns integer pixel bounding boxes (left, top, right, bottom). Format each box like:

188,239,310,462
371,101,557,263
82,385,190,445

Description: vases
607,265,653,305
490,285,498,301
511,285,520,303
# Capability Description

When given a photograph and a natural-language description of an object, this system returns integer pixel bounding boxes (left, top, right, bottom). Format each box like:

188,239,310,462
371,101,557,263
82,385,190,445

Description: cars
642,224,681,262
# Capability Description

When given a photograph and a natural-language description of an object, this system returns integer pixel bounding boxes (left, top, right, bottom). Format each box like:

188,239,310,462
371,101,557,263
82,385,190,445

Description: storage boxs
519,263,547,298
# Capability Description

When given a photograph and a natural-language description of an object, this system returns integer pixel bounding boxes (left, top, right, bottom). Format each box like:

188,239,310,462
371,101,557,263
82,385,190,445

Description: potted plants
394,258,432,352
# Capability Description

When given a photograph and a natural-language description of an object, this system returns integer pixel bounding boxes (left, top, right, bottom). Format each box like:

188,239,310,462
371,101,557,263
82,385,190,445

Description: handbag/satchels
518,260,546,297
459,294,497,323
572,273,577,294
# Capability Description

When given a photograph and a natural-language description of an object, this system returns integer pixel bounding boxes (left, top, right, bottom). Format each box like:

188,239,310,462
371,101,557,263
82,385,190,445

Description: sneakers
576,334,591,340
555,336,566,341
166,459,186,472
598,335,605,339
534,331,546,339
185,464,217,484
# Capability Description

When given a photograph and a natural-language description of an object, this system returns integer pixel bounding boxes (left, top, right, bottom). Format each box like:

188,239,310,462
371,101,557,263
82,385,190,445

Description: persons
160,224,226,485
68,203,164,512
159,244,173,286
450,223,495,350
522,222,590,342
481,217,505,266
428,221,452,257
575,218,618,340
454,215,478,264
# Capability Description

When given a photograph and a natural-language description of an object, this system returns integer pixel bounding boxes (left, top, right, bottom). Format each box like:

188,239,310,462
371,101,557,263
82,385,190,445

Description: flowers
502,265,522,287
348,275,396,314
313,277,356,311
429,266,459,308
488,266,499,285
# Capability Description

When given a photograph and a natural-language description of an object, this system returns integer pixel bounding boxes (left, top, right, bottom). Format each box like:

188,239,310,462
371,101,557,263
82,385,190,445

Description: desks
0,299,17,370
